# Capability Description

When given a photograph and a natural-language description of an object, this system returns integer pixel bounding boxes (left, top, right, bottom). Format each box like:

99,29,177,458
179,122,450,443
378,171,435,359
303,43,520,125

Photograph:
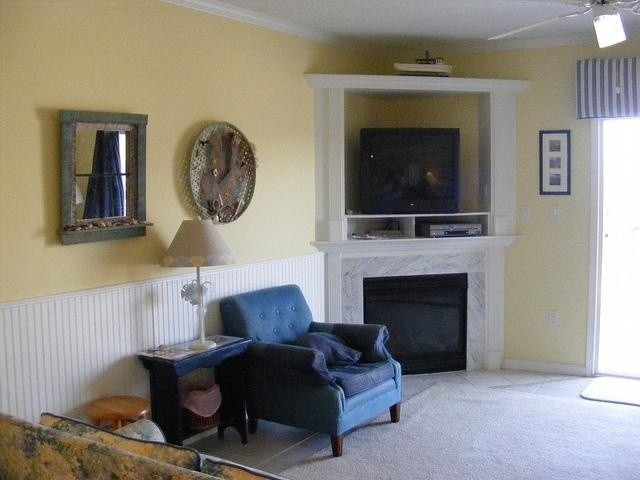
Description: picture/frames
539,130,572,195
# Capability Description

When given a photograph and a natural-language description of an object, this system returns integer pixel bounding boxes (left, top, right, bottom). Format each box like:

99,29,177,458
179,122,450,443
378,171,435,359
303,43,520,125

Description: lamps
592,1,627,49
159,219,236,349
75,183,84,205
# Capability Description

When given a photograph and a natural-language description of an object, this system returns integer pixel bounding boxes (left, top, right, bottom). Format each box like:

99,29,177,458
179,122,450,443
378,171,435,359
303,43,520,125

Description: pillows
37,412,200,471
198,458,285,480
294,332,362,367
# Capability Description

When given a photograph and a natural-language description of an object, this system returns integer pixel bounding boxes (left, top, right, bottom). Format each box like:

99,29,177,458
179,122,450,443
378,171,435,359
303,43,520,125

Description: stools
88,395,148,431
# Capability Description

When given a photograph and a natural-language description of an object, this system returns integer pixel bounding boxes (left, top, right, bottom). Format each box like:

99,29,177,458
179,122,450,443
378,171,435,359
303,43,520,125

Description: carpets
581,376,640,408
278,382,639,480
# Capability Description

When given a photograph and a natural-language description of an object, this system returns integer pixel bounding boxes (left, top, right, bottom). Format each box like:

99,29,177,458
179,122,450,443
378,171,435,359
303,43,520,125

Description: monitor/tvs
359,127,460,214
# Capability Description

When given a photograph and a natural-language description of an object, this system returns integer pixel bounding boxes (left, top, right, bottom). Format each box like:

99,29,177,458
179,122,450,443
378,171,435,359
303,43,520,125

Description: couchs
1,412,226,480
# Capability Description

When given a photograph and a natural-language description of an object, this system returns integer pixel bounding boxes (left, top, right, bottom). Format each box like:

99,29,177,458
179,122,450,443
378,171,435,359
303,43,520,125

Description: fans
484,0,640,41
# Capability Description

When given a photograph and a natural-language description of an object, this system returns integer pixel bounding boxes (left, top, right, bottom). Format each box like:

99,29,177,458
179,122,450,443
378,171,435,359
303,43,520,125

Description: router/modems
394,62,452,77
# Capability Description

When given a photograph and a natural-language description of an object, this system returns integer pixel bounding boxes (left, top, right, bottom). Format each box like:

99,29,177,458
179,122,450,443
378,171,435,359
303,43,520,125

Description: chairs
220,283,402,457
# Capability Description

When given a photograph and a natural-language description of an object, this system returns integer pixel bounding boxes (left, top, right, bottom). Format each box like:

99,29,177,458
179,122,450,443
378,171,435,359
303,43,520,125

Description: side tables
138,335,253,449
